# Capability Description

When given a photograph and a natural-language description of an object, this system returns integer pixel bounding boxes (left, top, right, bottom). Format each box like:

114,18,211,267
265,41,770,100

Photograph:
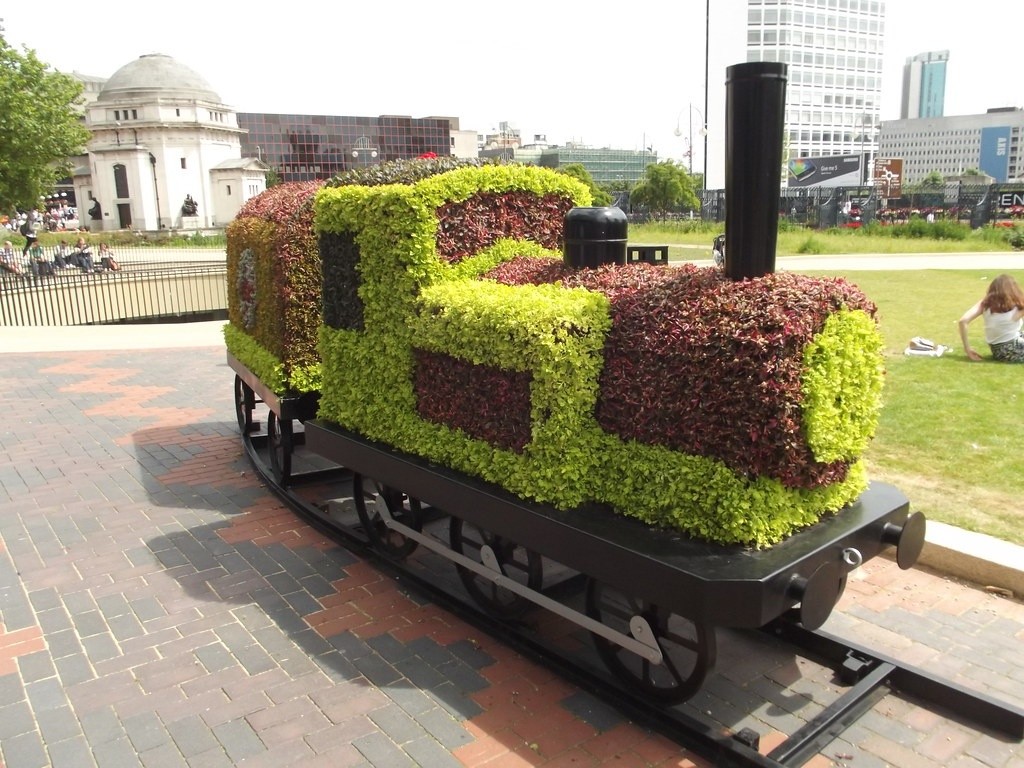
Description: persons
959,274,1024,363
791,205,797,219
183,194,198,215
88,198,102,219
0,201,121,280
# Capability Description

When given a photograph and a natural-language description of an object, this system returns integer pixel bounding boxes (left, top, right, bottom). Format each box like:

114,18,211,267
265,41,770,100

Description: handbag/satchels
910,336,938,351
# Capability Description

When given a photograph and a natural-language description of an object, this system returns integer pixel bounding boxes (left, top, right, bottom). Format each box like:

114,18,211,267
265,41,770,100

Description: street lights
350,137,379,169
675,104,708,192
491,122,519,163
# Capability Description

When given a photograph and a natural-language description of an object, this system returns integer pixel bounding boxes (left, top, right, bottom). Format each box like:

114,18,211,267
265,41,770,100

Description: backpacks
20,220,30,236
109,258,119,270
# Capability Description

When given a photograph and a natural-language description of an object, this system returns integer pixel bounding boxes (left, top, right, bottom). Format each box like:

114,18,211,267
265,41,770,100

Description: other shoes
65,263,76,270
87,269,94,273
